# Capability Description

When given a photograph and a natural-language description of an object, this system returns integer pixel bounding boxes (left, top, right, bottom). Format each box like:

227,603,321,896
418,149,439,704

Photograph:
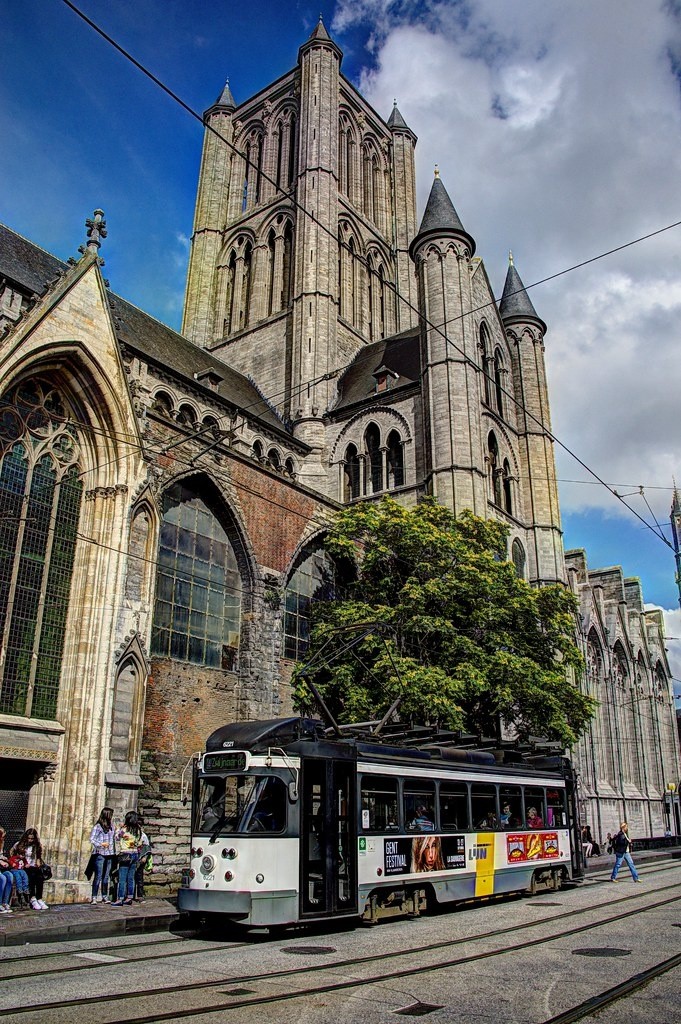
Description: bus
175,623,588,936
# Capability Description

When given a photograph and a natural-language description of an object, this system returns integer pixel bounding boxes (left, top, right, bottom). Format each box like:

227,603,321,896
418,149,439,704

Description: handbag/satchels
0,859,12,873
37,857,52,881
117,852,132,866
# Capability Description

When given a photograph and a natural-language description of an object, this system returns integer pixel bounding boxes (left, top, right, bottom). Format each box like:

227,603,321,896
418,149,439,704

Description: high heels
111,901,123,906
122,900,132,905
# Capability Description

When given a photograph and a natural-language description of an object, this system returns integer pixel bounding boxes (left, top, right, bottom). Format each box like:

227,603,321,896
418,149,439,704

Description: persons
90,807,153,906
252,784,276,830
611,822,643,884
409,804,434,831
580,825,617,858
0,826,50,914
476,802,545,829
664,827,672,845
410,836,446,873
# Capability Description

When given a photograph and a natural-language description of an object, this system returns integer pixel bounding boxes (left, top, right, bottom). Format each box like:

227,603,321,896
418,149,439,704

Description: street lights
667,782,678,846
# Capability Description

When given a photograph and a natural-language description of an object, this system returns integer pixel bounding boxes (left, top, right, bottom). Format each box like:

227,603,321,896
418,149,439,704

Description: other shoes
634,879,643,883
18,893,25,904
24,894,30,903
137,897,147,903
91,900,97,905
102,898,111,904
612,879,618,883
0,903,13,913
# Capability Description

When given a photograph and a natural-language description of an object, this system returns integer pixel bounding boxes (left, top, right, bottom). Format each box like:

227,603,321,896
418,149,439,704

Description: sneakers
30,898,42,910
37,900,49,909
